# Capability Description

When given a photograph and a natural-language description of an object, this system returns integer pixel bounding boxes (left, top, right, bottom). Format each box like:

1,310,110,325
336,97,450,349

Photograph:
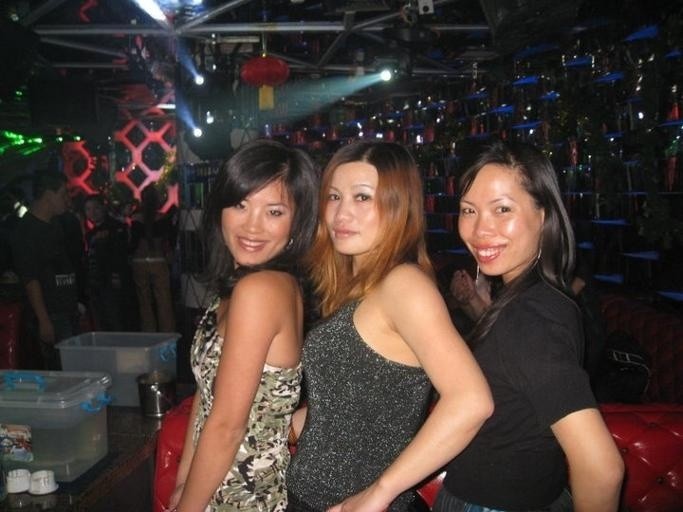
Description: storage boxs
54,332,182,407
1,369,111,481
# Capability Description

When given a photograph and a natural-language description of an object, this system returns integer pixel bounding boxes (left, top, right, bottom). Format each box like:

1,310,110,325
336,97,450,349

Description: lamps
379,67,395,82
206,114,214,126
193,126,204,140
194,73,205,87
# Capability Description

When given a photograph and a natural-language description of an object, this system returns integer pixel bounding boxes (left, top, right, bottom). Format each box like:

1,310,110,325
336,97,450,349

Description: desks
0,334,201,512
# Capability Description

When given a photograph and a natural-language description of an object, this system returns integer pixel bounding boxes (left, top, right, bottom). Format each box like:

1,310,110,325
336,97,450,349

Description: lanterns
242,56,290,112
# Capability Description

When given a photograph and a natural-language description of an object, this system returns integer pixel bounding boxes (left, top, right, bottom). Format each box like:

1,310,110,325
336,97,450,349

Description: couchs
151,393,683,512
579,283,683,403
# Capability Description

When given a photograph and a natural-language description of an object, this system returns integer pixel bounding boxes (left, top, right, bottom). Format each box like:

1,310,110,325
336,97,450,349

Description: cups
31,471,55,492
6,469,30,493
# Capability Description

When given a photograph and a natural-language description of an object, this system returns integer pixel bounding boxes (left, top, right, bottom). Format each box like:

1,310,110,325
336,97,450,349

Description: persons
433,141,626,512
0,167,167,368
286,141,495,512
168,140,322,512
571,273,652,408
